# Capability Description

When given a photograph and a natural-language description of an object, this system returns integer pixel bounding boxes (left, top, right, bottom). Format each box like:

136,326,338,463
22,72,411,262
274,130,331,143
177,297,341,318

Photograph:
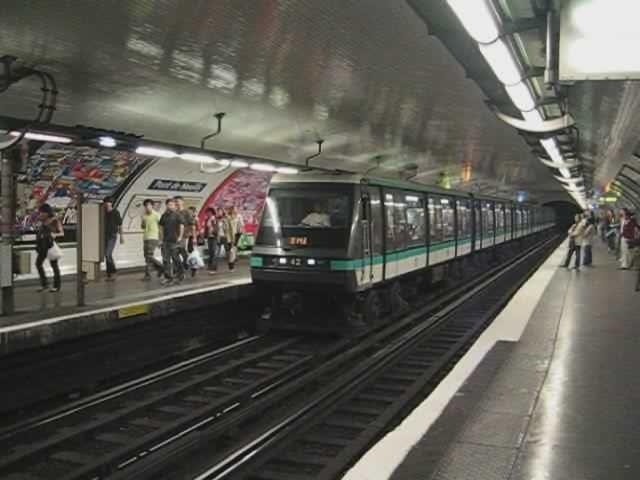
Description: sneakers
48,287,61,292
105,265,238,289
36,286,49,292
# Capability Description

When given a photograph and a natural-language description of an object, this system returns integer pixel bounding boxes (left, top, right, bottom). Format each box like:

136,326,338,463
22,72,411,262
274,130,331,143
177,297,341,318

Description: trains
251,170,557,339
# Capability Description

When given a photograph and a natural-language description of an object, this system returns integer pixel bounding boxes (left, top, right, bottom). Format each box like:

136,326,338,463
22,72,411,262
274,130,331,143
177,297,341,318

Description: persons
377,198,640,273
101,198,125,279
140,196,243,286
298,197,331,233
34,203,67,294
